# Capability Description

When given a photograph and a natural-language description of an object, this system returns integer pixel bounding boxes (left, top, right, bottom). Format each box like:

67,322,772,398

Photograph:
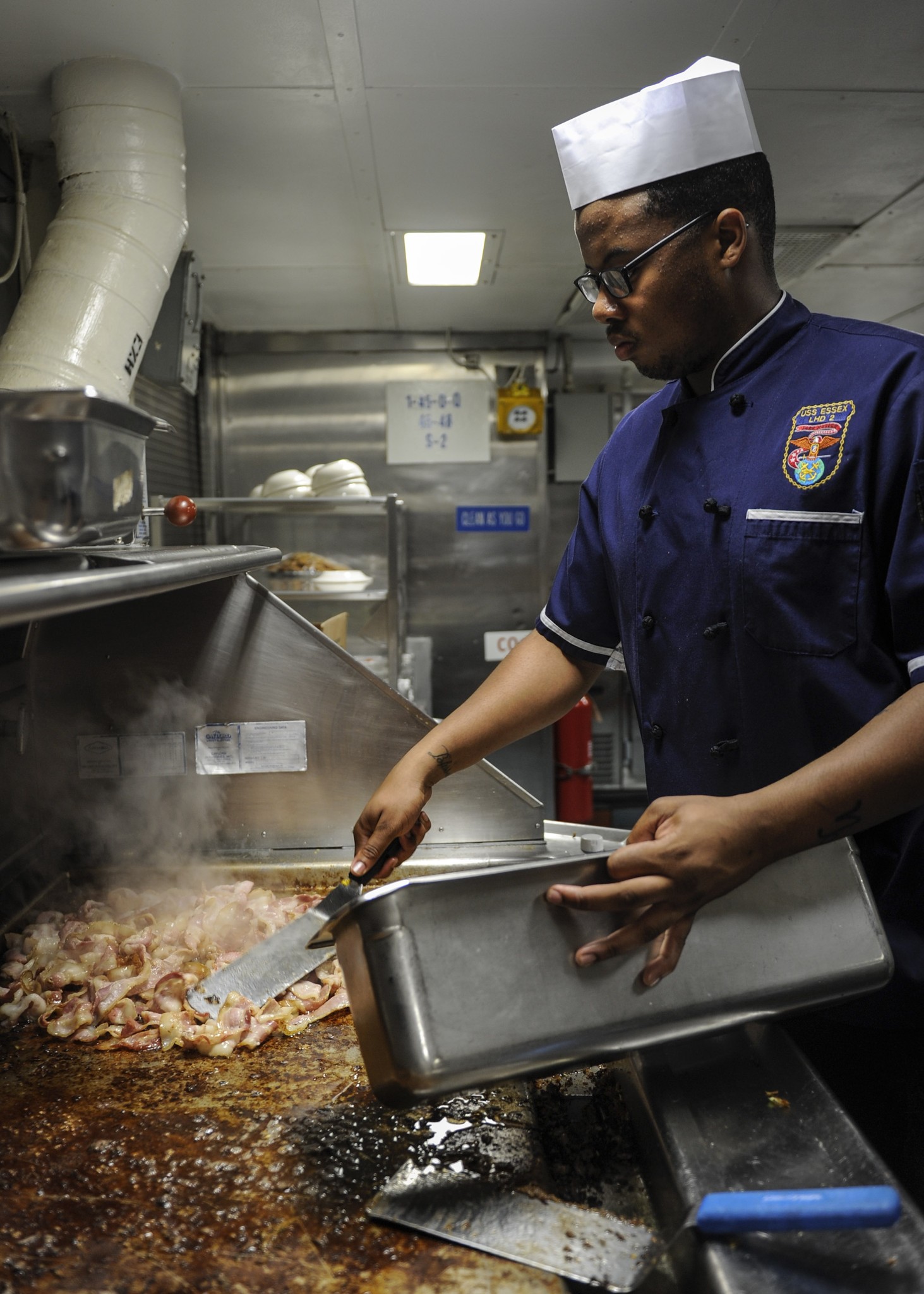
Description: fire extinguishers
555,694,594,823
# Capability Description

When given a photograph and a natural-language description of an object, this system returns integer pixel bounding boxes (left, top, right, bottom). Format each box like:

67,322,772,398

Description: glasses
573,211,750,305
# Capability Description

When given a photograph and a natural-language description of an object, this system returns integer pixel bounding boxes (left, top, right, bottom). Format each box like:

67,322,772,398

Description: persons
350,56,924,1214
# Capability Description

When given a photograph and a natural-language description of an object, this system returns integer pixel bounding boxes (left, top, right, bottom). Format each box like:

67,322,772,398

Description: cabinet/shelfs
150,494,414,692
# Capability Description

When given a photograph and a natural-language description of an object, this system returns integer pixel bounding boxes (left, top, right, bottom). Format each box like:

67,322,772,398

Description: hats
550,54,763,210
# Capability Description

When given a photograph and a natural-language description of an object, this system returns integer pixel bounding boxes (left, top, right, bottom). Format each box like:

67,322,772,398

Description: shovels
189,839,402,1018
366,1156,904,1293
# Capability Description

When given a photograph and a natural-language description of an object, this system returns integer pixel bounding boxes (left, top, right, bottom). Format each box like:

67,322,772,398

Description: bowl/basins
312,571,373,591
248,458,369,500
